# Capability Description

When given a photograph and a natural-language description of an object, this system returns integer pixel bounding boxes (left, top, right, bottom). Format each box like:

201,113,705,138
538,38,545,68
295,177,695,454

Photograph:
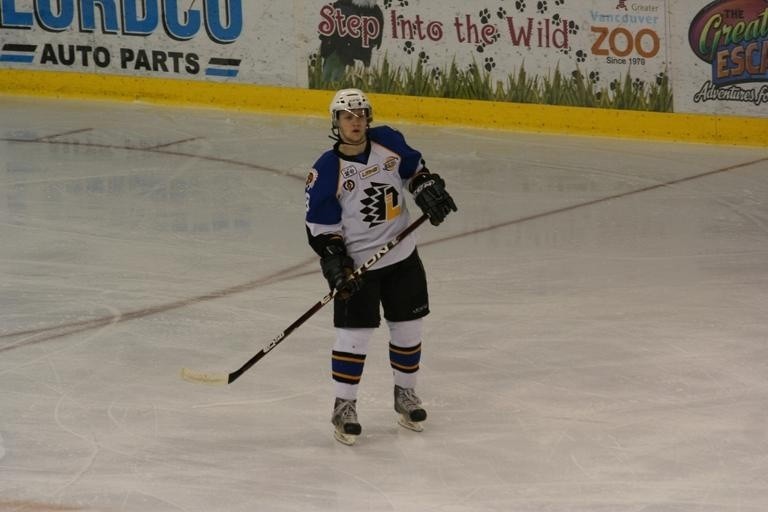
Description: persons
303,87,457,436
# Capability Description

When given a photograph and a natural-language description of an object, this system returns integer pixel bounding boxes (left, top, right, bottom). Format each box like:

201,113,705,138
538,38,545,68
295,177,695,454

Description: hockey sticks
182,214,429,385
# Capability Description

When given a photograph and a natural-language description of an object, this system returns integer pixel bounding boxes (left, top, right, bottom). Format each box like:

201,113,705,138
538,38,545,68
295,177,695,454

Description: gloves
409,168,457,227
320,243,364,301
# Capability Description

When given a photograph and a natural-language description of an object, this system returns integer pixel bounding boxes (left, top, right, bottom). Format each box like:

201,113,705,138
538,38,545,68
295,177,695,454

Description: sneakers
394,384,426,422
331,397,361,435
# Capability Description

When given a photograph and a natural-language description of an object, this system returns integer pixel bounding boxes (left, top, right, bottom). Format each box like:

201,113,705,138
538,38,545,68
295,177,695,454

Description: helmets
329,88,372,126
